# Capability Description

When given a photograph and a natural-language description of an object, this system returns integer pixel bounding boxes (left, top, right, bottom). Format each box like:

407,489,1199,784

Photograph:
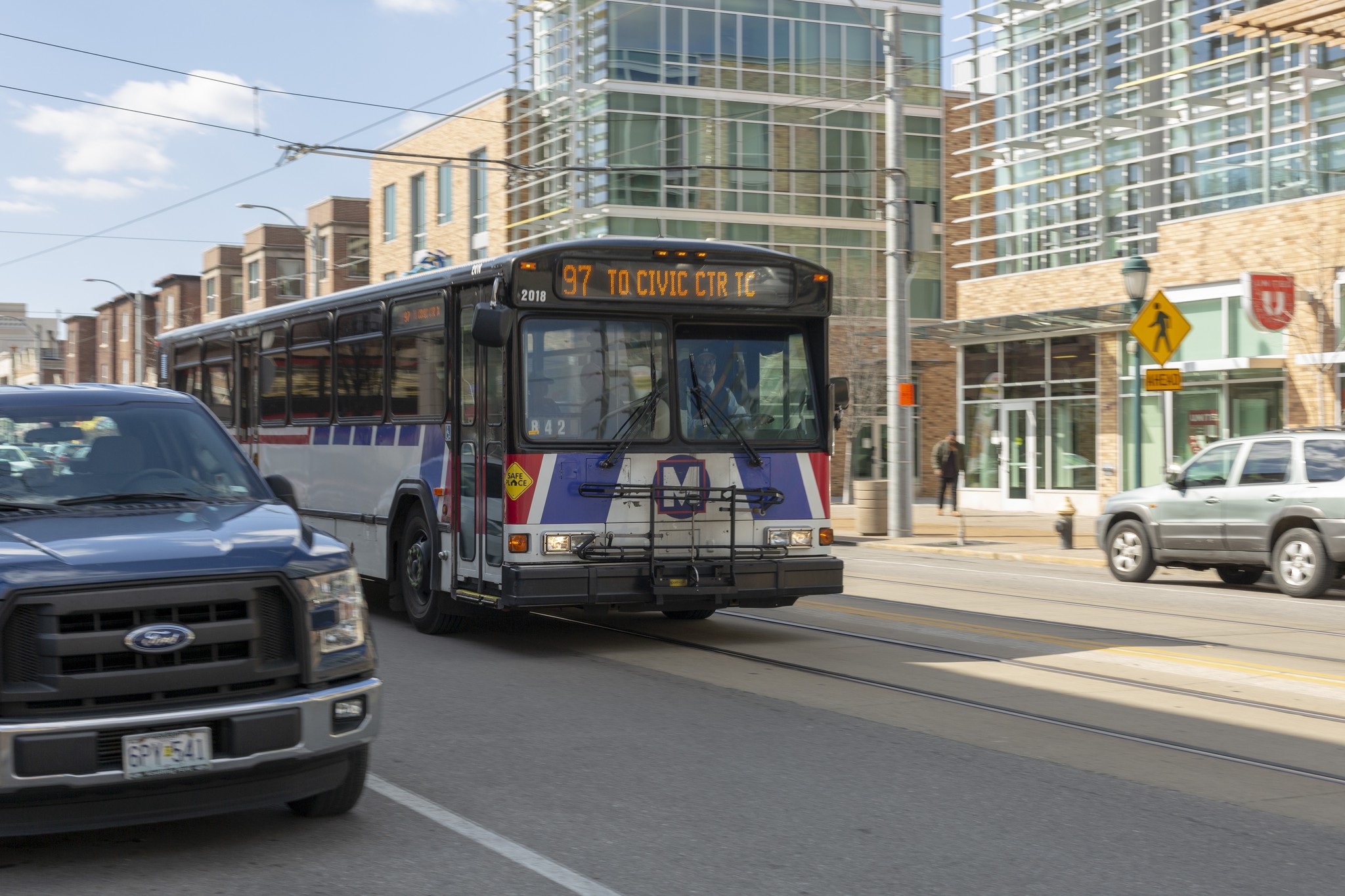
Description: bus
152,237,845,632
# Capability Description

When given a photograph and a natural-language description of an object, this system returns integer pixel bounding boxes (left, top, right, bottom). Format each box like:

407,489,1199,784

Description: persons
931,430,964,516
580,363,625,439
528,372,561,417
686,344,768,439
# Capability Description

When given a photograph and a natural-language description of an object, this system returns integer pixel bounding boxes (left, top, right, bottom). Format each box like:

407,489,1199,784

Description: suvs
1093,425,1345,599
0,384,381,819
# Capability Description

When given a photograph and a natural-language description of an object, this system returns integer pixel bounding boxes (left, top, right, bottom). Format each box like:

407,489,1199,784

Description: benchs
0,460,91,496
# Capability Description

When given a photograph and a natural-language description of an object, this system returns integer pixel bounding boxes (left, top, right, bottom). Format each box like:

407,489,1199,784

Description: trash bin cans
852,479,888,535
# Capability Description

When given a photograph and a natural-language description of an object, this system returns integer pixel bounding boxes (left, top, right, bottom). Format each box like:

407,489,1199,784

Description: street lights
85,277,163,339
236,200,323,303
1120,247,1154,487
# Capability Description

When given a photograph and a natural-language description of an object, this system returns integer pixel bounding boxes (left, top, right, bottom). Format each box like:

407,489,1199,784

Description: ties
704,384,712,396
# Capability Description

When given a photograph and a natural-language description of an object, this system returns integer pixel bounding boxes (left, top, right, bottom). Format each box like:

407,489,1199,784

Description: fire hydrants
1055,496,1077,550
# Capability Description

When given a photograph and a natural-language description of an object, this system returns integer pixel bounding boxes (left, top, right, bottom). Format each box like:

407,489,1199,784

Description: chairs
87,436,154,493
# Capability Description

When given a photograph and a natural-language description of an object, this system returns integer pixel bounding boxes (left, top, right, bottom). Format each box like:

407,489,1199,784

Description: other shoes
936,509,943,515
951,511,960,516
